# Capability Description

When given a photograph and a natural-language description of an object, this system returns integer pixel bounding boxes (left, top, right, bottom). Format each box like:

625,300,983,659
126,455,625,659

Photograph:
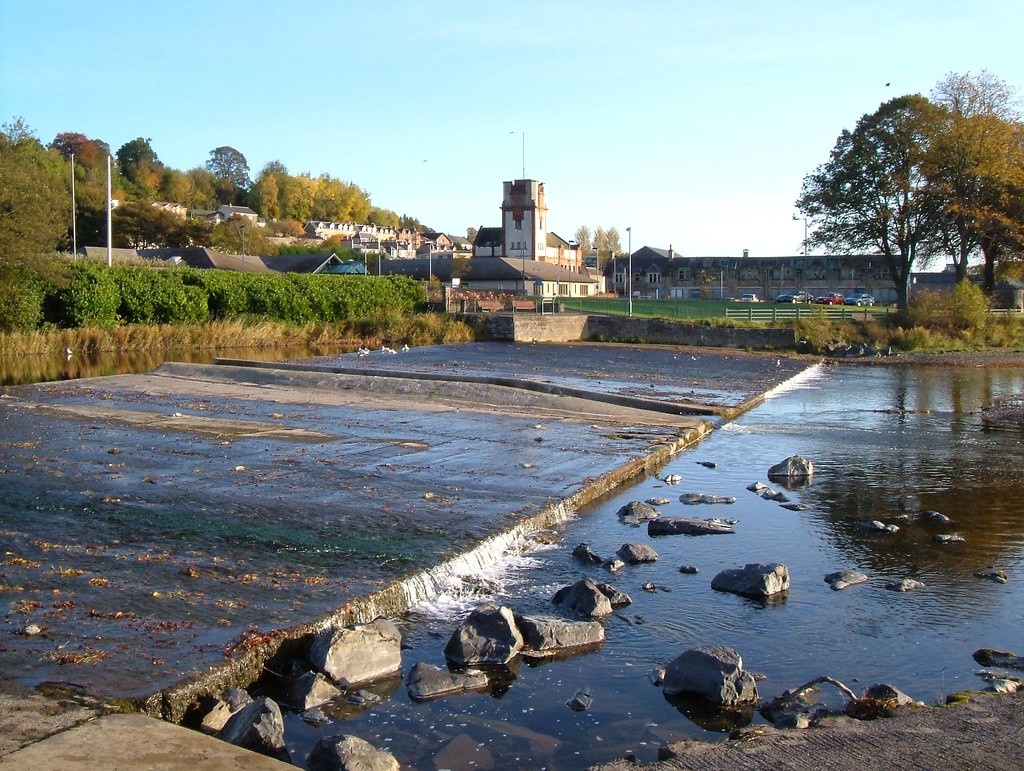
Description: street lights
509,130,526,180
624,227,634,319
240,223,246,273
793,213,808,304
592,247,601,300
567,240,575,297
429,243,435,286
68,153,78,262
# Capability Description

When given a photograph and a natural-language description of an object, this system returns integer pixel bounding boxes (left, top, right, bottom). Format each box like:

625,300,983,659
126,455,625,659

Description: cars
775,290,814,305
845,293,876,307
640,296,651,299
815,292,845,306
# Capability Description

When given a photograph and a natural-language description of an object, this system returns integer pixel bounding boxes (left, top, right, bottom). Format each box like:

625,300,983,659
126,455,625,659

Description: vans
740,293,761,302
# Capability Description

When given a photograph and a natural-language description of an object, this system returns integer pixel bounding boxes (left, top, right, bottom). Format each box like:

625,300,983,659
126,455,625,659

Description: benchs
477,300,503,312
851,313,876,322
511,301,537,312
